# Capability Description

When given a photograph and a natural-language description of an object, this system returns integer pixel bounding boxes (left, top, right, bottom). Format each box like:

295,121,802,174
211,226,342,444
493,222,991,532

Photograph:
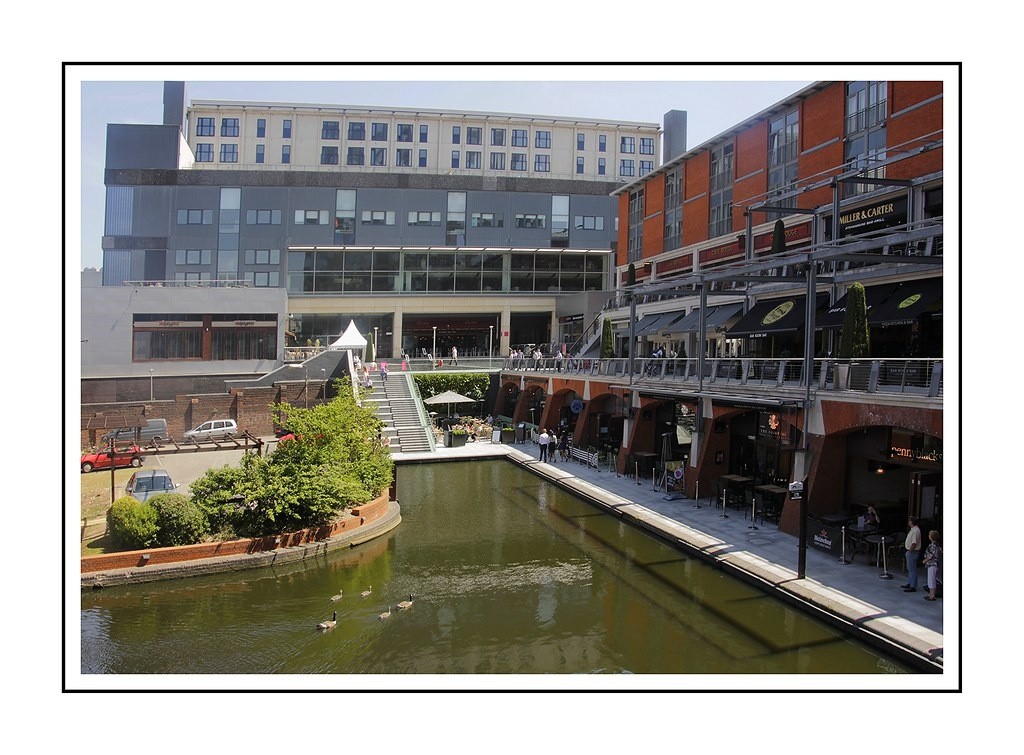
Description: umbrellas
423,390,476,416
307,338,319,352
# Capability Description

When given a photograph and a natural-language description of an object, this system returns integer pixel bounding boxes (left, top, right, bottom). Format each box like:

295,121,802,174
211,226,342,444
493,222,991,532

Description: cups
870,508,874,512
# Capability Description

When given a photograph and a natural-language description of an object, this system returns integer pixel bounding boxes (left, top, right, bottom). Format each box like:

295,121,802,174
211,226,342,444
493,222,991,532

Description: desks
721,475,752,509
633,452,658,476
821,513,893,564
753,485,788,525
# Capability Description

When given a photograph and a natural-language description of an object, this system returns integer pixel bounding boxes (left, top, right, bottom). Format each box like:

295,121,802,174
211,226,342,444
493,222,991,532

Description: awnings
815,275,943,334
285,330,297,340
617,309,684,338
667,302,745,334
725,290,830,339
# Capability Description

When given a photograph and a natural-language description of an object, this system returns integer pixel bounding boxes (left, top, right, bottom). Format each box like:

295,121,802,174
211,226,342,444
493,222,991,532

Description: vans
100,418,170,448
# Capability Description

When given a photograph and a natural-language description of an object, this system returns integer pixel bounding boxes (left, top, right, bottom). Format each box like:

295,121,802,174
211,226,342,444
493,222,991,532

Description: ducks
330,589,343,601
396,594,413,608
360,585,372,598
316,611,337,629
377,605,392,619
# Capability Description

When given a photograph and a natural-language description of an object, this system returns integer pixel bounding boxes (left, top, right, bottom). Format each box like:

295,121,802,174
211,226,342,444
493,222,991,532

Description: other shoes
566,458,568,462
554,459,556,463
544,460,547,463
924,596,936,601
539,459,542,461
904,587,917,592
548,460,551,462
560,460,563,462
901,584,910,588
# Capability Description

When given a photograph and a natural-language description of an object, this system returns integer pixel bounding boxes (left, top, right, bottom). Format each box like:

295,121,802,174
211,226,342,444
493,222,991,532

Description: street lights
150,368,154,401
489,325,494,370
321,368,326,403
374,327,380,358
432,327,437,371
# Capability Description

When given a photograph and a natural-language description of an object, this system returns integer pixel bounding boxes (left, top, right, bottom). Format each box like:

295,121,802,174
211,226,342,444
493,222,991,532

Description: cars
274,428,326,450
124,469,181,503
183,418,239,445
80,440,147,473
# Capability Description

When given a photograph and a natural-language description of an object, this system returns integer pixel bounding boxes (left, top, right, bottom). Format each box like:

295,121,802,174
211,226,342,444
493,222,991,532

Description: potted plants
452,430,466,447
502,428,515,445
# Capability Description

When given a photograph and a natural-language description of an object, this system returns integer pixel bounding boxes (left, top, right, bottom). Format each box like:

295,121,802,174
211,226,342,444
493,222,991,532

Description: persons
353,352,373,394
863,503,880,525
922,530,942,601
454,412,459,418
477,414,493,424
902,513,920,592
509,349,563,373
647,345,666,373
511,345,546,354
538,428,572,463
380,365,388,380
462,421,478,442
435,346,458,368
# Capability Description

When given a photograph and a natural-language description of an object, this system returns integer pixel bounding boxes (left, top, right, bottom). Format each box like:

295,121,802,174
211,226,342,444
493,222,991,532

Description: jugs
858,516,864,529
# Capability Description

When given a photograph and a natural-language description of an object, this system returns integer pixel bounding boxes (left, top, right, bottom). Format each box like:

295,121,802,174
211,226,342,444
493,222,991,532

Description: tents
329,319,367,362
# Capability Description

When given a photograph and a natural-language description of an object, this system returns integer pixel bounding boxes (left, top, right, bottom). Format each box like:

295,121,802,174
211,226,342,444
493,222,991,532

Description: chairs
887,533,909,572
